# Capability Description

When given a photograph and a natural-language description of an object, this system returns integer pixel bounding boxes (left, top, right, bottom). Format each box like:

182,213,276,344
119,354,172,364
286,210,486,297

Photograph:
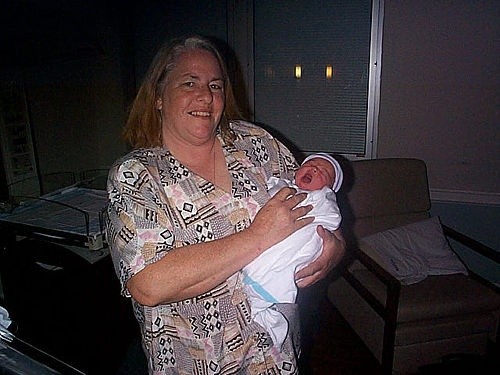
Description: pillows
355,216,468,285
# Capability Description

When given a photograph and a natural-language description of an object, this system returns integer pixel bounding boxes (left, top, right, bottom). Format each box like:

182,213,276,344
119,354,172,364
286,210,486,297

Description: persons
104,37,347,375
242,152,343,353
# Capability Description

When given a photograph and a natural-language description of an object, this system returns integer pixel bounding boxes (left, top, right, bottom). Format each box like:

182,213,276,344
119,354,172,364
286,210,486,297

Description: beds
0,168,111,257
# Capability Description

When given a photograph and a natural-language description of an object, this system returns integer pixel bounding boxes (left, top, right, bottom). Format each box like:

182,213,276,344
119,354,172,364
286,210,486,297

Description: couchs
323,158,500,375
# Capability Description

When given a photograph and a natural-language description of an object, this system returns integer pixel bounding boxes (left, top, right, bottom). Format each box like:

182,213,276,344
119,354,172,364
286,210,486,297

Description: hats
301,152,343,192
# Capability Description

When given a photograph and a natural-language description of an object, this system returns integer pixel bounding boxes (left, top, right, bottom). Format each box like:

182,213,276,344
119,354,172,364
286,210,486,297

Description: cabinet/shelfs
0,80,41,206
